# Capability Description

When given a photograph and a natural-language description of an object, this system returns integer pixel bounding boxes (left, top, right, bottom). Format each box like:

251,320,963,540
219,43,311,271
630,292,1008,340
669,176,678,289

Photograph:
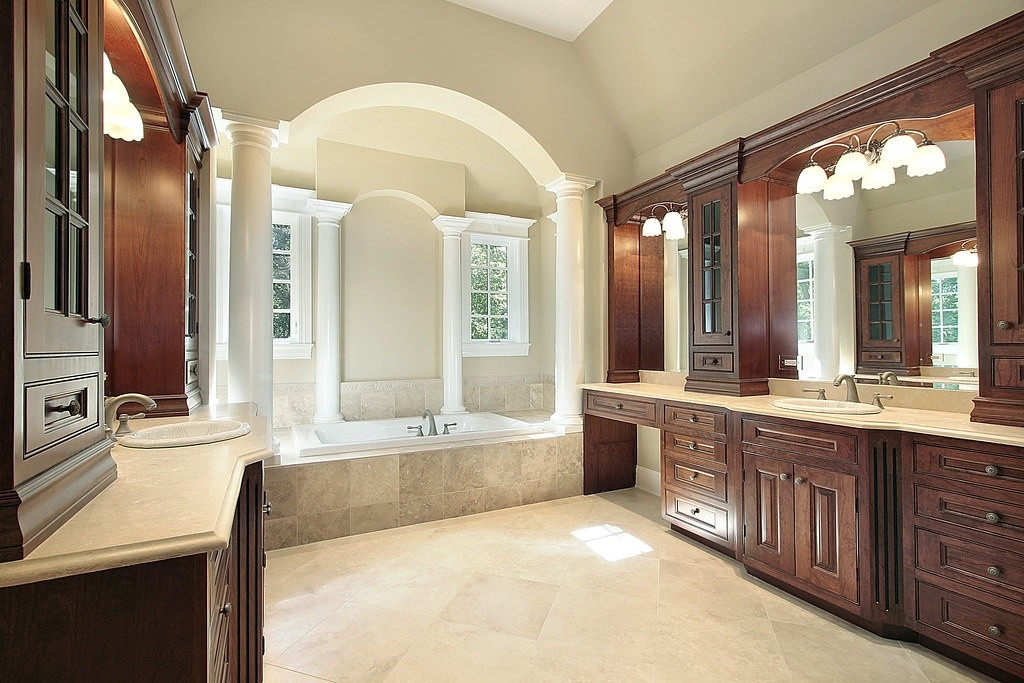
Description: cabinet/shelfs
688,179,738,380
5,1,106,487
184,123,206,413
975,78,1023,396
658,402,737,550
204,460,265,683
739,417,872,621
855,255,905,369
899,430,1024,679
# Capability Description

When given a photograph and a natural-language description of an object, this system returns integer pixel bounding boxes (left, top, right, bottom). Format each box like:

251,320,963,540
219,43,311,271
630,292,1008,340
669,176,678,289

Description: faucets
104,392,158,442
422,408,439,436
877,371,899,385
833,373,861,403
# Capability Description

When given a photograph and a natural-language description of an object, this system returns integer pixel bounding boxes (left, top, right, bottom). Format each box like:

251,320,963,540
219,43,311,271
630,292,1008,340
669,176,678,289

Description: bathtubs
292,411,544,457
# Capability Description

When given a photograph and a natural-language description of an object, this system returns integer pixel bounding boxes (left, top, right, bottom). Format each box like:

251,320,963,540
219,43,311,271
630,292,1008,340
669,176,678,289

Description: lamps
797,122,946,200
952,238,979,268
102,49,145,142
643,202,688,241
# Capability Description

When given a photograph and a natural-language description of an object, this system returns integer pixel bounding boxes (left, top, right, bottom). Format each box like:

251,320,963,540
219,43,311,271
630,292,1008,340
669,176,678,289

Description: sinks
118,419,251,448
948,376,979,381
771,398,882,414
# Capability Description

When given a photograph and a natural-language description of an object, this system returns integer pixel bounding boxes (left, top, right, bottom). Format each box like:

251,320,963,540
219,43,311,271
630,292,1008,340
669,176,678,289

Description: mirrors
917,239,979,369
639,208,689,374
767,103,980,388
